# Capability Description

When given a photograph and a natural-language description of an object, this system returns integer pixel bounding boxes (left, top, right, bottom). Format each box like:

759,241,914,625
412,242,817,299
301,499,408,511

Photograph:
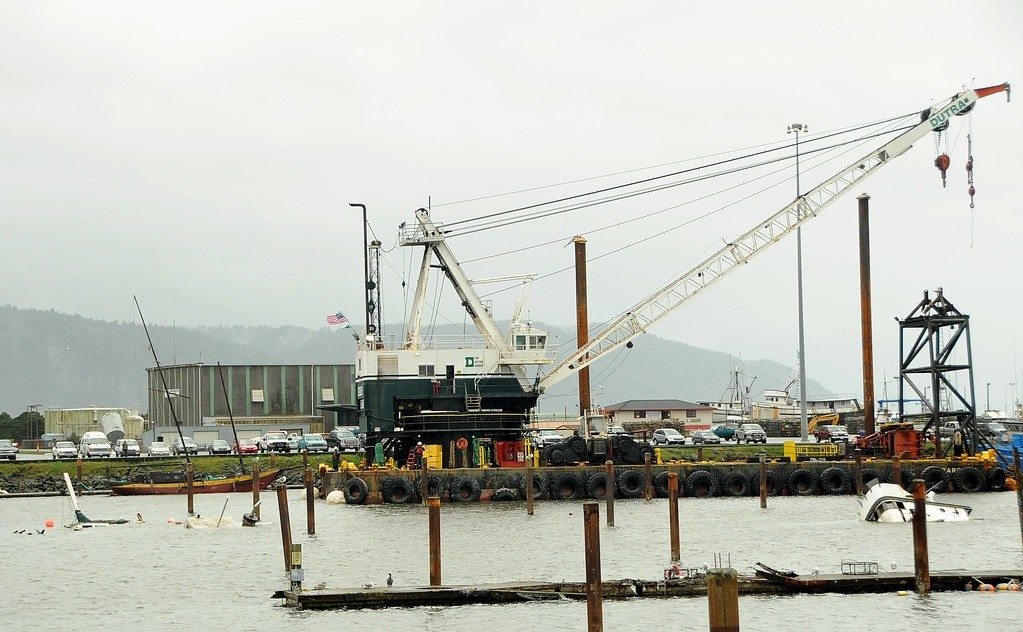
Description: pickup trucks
324,430,366,452
813,425,849,444
735,423,767,445
710,423,737,442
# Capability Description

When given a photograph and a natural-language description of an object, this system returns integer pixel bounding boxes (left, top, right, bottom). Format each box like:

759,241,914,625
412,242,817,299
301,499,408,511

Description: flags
326,312,347,325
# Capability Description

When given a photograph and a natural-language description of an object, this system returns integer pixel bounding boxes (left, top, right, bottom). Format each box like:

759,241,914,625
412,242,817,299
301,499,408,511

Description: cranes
346,81,1011,469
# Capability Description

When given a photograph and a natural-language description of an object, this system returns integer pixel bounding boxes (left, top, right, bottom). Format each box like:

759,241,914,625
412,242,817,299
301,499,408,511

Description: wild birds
812,567,818,579
362,583,374,590
387,573,393,586
314,582,327,595
890,561,896,573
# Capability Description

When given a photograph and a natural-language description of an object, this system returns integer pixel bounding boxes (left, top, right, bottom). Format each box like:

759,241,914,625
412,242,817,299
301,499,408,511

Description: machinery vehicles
779,412,839,437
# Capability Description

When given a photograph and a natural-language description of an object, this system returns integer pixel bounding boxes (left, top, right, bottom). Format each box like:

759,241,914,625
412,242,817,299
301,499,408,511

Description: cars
297,433,328,453
607,426,629,437
692,430,721,445
652,428,686,445
0,439,19,460
147,441,171,456
232,437,259,454
208,440,232,455
51,441,78,459
859,411,1006,442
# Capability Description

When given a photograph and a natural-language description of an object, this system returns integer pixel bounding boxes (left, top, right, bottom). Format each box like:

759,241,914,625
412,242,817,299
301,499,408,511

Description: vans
113,438,141,457
79,431,112,458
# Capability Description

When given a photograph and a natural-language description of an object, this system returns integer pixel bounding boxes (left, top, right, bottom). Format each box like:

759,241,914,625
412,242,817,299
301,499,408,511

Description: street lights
26,403,42,440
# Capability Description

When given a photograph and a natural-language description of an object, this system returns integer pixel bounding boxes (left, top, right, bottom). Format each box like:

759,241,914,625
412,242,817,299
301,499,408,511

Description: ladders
407,447,415,470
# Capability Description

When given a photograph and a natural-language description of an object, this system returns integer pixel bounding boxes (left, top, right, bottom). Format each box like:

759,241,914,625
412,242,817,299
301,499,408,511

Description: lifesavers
618,469,646,498
818,466,850,495
585,472,617,499
456,438,468,449
919,465,985,494
519,473,548,500
786,468,818,496
720,471,751,497
382,476,414,504
415,474,445,503
344,477,368,504
653,471,683,498
987,466,1006,492
449,474,481,501
684,470,720,498
549,472,582,500
885,468,915,494
851,468,881,494
369,325,376,333
751,469,784,497
490,488,520,501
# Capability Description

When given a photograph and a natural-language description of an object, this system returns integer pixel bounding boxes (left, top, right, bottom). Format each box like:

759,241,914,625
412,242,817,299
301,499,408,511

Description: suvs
172,437,199,455
252,430,291,454
532,427,564,449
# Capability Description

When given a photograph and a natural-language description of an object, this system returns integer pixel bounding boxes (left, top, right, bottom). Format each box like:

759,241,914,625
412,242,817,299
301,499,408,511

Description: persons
122,441,128,457
333,449,341,463
414,441,425,469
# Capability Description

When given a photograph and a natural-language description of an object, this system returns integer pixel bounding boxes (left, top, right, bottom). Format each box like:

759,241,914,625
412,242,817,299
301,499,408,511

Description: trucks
286,432,302,450
